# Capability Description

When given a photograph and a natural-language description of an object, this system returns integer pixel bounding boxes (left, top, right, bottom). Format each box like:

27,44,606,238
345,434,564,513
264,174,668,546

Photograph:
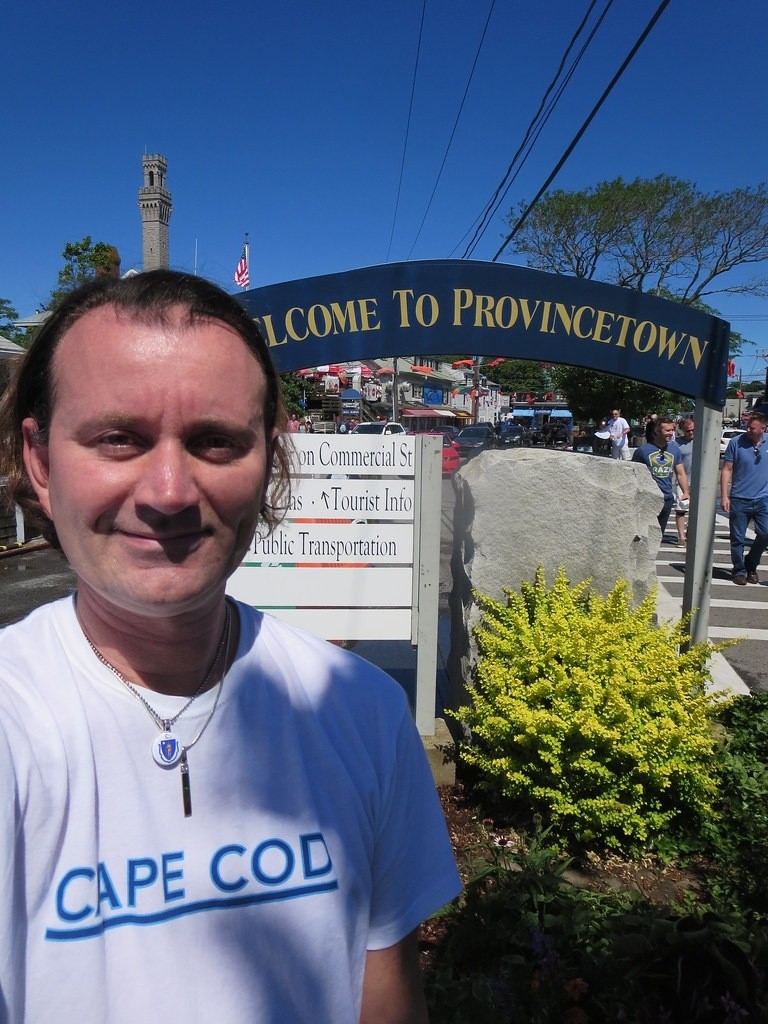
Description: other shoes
747,568,759,584
676,539,686,548
732,574,747,585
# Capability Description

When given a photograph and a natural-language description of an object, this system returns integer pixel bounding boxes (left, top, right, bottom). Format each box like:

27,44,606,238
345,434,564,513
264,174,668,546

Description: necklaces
73,596,234,816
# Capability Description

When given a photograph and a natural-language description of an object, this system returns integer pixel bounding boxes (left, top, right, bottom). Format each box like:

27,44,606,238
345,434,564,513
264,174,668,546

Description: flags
233,243,249,288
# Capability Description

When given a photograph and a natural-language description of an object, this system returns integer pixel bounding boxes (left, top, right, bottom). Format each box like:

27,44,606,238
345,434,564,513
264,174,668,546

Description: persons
541,420,549,448
2,270,466,1023
644,416,650,423
550,420,559,447
674,419,694,548
721,411,768,586
632,419,690,537
288,412,388,434
606,409,633,461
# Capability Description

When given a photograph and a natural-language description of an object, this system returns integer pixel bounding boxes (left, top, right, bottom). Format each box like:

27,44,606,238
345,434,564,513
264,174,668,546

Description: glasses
612,413,618,416
685,428,694,433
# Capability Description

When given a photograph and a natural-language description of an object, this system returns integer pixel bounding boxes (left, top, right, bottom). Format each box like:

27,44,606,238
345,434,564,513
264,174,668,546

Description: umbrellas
292,359,374,377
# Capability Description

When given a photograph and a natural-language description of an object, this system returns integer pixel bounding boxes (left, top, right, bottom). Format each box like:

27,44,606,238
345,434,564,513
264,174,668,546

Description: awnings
512,407,572,417
402,409,475,418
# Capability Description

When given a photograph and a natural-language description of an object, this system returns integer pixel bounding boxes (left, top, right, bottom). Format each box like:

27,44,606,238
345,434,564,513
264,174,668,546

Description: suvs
350,421,407,436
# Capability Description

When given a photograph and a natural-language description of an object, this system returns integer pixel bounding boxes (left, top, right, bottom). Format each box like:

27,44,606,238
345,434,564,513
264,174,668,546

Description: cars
403,422,569,478
720,429,747,458
